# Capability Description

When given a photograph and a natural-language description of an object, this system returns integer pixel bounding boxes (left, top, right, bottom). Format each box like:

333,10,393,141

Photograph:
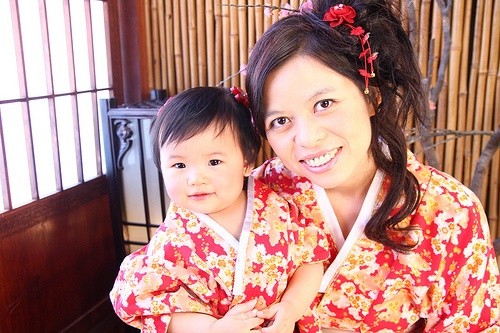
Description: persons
104,79,329,333
227,1,500,333
